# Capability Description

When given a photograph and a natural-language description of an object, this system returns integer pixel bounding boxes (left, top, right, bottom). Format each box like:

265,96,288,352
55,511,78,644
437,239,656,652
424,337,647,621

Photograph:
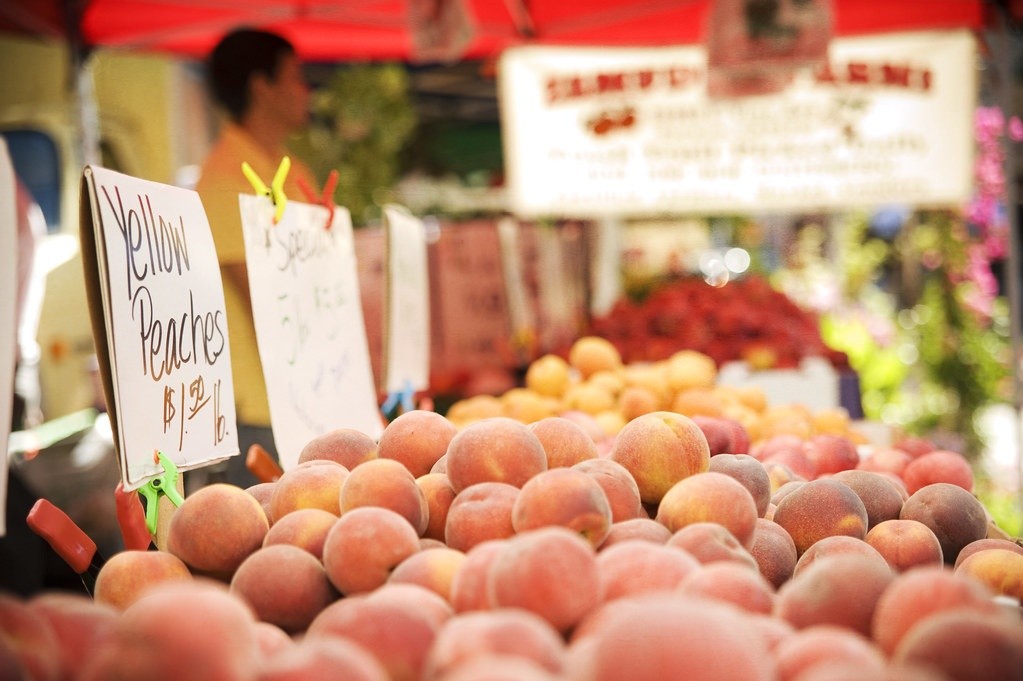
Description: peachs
0,338,1023,681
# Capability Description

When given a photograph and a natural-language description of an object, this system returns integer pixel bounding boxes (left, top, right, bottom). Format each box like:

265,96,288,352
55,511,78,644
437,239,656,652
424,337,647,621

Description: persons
194,24,322,490
